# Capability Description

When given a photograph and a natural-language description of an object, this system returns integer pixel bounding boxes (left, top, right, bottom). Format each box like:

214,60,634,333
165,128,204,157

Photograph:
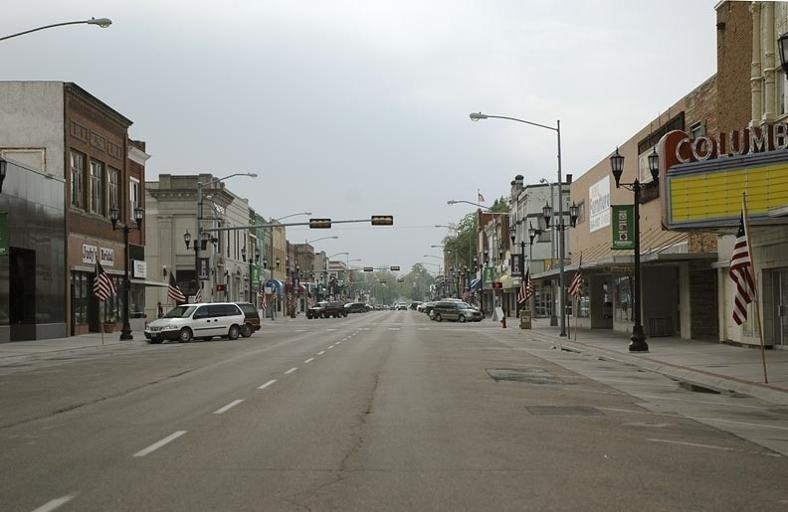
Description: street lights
0,17,112,42
196,172,259,301
269,212,311,320
610,144,662,351
304,236,361,269
109,201,144,340
182,230,217,303
434,112,579,337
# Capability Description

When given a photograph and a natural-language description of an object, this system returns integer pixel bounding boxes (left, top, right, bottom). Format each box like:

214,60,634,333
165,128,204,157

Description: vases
104,323,116,332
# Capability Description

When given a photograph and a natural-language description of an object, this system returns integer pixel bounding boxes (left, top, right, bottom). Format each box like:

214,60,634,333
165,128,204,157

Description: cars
144,302,261,343
303,296,485,323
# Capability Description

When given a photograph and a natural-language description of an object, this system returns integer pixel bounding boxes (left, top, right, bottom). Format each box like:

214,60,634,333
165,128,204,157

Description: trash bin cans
520,310,531,329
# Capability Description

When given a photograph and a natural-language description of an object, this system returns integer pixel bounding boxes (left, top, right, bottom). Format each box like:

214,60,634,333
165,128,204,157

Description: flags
168,272,186,302
517,266,535,304
568,264,584,306
195,288,202,303
93,261,117,301
729,206,756,325
